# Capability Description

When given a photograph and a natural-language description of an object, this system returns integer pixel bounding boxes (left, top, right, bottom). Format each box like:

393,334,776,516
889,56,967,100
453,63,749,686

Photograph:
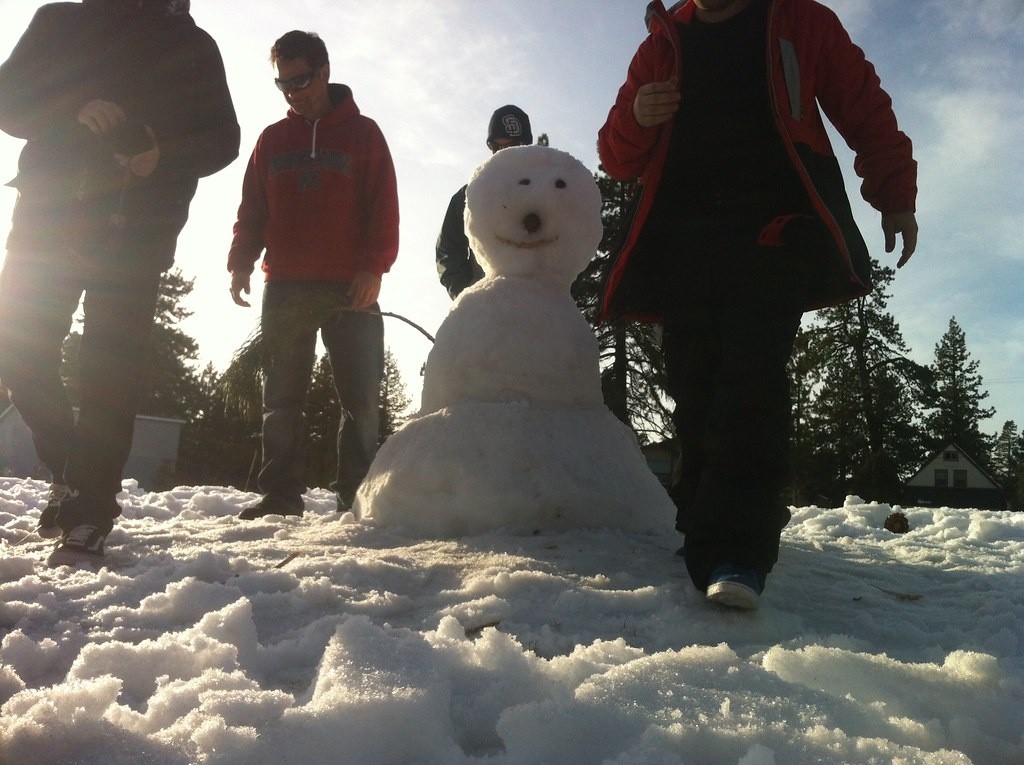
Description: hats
487,105,532,143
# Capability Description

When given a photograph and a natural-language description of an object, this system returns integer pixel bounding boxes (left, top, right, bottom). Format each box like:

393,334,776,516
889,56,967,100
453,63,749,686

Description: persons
598,0,917,609
0,0,240,567
229,31,399,520
436,106,532,300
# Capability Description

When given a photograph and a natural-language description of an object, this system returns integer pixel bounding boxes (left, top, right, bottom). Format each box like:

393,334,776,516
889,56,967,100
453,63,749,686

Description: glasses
276,70,317,89
487,142,520,150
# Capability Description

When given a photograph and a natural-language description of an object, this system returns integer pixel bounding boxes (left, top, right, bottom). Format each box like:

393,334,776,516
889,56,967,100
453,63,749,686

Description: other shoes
239,493,304,519
706,563,762,611
336,501,355,513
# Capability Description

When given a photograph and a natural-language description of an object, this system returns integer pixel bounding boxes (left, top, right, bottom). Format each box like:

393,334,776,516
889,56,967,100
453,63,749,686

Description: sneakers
49,523,105,572
38,483,79,528
